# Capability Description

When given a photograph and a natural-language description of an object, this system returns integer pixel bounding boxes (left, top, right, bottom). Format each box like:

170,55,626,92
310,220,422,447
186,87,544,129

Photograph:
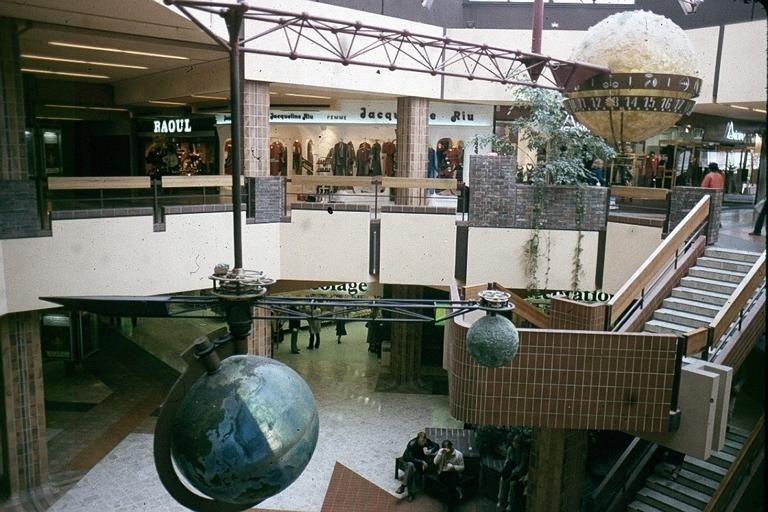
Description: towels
381,341,391,366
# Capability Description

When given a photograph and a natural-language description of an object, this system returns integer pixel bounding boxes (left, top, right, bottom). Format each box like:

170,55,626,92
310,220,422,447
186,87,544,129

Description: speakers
456,487,465,500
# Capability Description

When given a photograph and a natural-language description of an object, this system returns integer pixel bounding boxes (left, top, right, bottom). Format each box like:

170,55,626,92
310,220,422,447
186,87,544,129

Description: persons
426,138,464,196
364,306,384,365
393,430,440,503
267,137,397,178
620,145,634,186
662,449,687,482
432,439,466,511
589,159,607,188
288,303,302,354
331,304,348,345
746,197,767,238
161,143,180,195
697,162,724,228
725,365,746,433
494,433,525,511
306,299,323,349
144,141,160,175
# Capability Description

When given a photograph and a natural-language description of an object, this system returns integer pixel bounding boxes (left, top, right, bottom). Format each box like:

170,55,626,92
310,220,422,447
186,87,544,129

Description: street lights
501,461,527,481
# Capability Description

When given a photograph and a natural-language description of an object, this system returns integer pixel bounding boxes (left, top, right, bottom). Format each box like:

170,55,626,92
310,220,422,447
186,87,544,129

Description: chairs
506,505,512,511
749,232,761,235
396,487,404,494
408,495,413,502
307,347,313,349
293,349,301,354
497,502,501,507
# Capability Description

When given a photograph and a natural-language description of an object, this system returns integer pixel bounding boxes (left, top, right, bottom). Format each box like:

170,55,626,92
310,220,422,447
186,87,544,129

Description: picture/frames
426,428,480,459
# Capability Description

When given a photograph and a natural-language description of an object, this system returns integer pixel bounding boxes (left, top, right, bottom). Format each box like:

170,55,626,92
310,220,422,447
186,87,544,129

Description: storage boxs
478,456,530,493
396,458,476,512
